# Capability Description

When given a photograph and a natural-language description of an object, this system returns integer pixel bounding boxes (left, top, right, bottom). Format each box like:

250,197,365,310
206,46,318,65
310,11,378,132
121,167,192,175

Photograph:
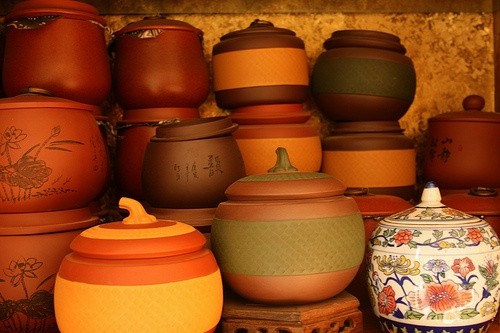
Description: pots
2,0,499,333
